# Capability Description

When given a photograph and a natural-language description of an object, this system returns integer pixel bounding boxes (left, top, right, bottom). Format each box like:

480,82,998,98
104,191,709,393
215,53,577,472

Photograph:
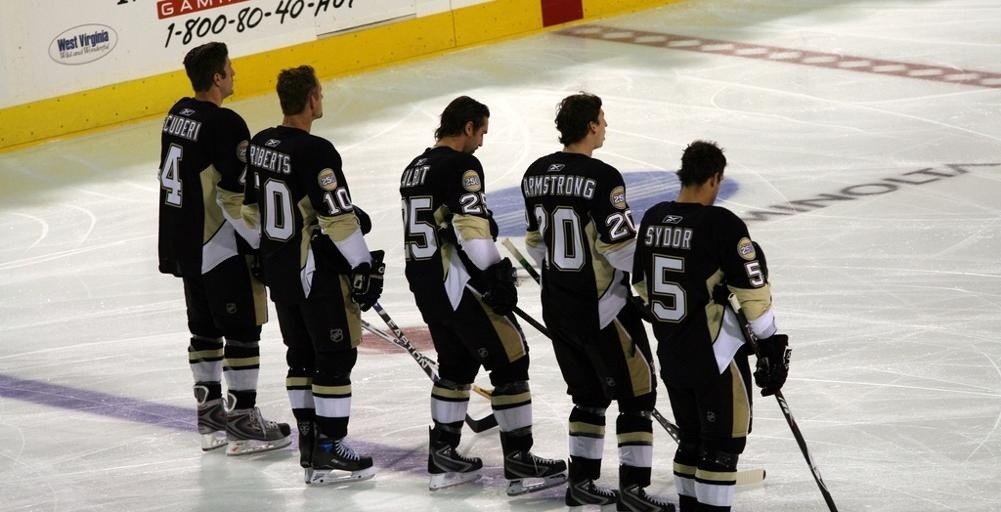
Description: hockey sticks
372,301,498,434
501,237,765,485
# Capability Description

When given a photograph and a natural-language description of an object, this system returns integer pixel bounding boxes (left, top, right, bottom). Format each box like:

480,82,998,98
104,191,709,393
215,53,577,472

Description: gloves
754,335,792,396
352,250,385,311
484,257,518,315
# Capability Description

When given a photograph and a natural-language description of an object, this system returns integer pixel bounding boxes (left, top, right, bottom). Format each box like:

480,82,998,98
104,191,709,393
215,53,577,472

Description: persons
241,65,384,471
159,42,291,443
521,93,677,511
399,96,566,480
631,141,792,512
